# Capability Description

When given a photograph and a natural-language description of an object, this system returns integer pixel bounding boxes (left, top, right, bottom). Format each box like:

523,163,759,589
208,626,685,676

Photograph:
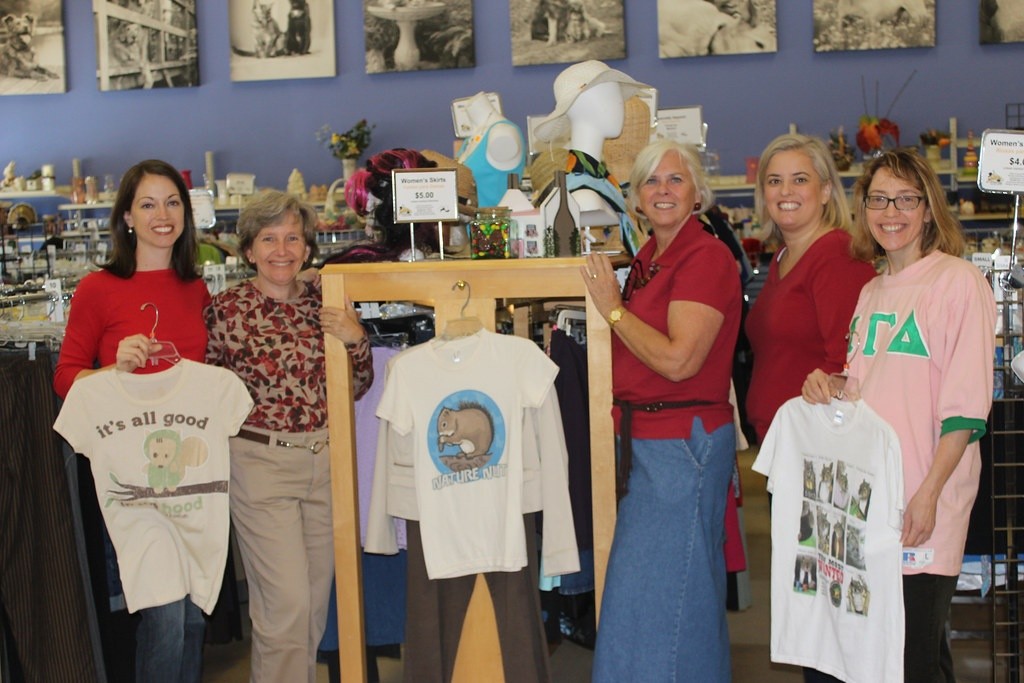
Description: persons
801,149,999,683
580,142,742,683
52,159,213,683
744,133,876,519
202,190,374,683
532,60,653,256
454,90,526,237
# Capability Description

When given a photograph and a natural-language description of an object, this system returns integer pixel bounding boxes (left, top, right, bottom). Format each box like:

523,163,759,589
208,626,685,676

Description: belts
237,427,330,455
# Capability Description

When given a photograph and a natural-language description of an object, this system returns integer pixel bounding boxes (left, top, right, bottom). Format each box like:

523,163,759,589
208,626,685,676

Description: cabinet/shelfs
0,190,117,241
955,175,1024,220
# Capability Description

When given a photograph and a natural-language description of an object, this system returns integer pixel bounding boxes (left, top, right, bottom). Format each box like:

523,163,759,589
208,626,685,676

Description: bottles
740,219,762,274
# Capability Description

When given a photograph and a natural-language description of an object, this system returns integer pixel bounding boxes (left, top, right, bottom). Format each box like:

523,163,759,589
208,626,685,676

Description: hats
419,147,511,217
532,59,657,144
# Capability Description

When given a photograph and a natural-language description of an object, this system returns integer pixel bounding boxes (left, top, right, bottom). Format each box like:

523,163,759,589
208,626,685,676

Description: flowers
316,119,376,159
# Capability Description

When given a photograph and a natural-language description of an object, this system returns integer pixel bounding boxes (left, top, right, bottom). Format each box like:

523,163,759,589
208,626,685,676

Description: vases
343,160,355,186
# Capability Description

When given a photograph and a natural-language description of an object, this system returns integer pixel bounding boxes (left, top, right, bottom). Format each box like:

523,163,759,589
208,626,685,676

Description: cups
925,145,941,159
745,157,759,183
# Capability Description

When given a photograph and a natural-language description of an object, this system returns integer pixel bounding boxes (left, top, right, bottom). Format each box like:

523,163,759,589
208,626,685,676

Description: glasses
863,192,926,211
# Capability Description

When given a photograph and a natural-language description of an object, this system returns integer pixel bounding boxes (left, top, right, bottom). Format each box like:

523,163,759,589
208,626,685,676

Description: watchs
607,306,626,327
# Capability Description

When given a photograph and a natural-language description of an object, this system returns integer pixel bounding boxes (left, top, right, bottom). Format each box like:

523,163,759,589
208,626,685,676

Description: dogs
110,22,141,65
835,0,930,37
531,0,617,48
0,13,59,80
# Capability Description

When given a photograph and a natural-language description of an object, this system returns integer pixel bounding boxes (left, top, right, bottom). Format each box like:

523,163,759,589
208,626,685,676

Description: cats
231,0,312,58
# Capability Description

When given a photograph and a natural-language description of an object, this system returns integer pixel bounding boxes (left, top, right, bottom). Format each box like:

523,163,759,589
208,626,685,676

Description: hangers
828,331,861,408
116,303,184,379
441,281,482,341
359,321,407,352
0,291,74,352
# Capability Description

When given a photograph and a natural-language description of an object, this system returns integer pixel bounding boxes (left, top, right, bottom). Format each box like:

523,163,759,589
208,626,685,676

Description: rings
592,275,597,279
326,322,329,327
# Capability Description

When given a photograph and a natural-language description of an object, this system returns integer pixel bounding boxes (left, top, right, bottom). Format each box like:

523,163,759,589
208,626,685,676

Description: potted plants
856,71,921,164
